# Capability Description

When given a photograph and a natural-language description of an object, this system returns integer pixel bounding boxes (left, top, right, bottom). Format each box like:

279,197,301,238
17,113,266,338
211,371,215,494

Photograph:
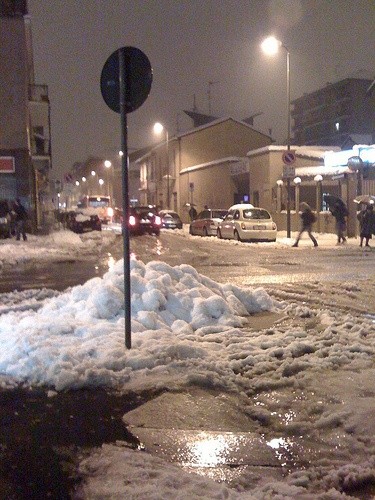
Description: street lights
259,36,291,238
154,121,170,209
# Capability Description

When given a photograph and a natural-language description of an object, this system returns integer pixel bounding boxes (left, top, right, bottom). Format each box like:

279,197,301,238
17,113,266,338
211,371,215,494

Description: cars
158,210,183,229
60,207,101,234
216,207,278,242
189,209,228,236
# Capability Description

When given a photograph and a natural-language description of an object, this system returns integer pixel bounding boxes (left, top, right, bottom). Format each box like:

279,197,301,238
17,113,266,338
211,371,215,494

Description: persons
291,202,318,247
188,203,197,221
356,202,375,247
0,198,29,241
331,200,347,246
196,205,211,219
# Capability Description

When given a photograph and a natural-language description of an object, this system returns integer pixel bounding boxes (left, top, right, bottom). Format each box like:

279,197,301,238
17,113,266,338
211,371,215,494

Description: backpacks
310,213,316,223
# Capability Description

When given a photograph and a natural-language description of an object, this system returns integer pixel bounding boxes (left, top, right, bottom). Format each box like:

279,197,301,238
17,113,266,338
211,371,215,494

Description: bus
80,194,113,225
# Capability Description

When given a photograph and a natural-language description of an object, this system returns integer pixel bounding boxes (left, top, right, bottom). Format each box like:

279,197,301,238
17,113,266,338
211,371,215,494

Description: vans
128,205,162,237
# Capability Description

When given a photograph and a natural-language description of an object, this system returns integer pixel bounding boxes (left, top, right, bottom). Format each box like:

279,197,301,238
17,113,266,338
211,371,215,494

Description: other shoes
336,242,341,245
292,243,298,248
342,240,347,244
313,243,318,247
365,244,370,248
359,246,362,248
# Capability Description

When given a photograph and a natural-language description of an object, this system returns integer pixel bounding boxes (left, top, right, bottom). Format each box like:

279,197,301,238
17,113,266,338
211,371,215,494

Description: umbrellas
323,194,346,208
354,195,375,204
